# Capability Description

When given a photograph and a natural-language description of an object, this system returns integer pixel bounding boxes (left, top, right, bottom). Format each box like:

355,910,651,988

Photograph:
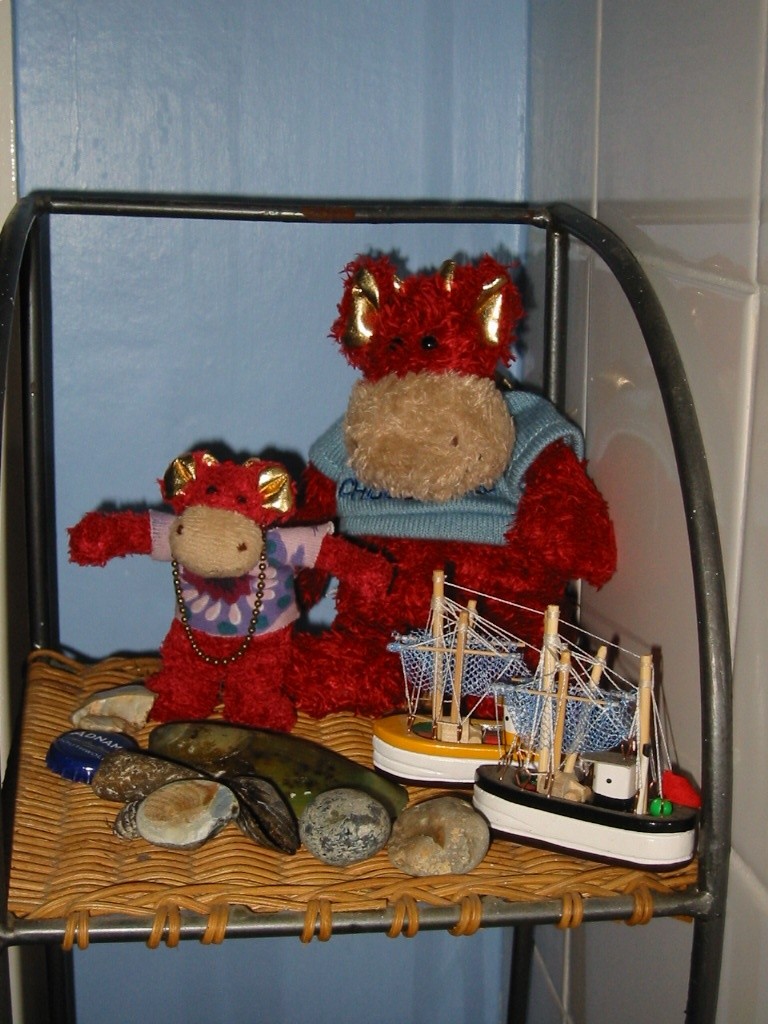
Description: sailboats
370,572,699,868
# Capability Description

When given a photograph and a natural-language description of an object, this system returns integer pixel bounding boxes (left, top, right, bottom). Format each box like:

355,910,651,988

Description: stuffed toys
68,448,394,731
281,251,619,718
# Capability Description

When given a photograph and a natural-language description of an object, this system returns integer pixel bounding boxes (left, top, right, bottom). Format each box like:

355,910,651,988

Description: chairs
0,185,732,1024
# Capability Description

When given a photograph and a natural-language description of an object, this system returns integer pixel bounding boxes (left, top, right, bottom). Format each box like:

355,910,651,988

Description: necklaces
171,525,268,665
